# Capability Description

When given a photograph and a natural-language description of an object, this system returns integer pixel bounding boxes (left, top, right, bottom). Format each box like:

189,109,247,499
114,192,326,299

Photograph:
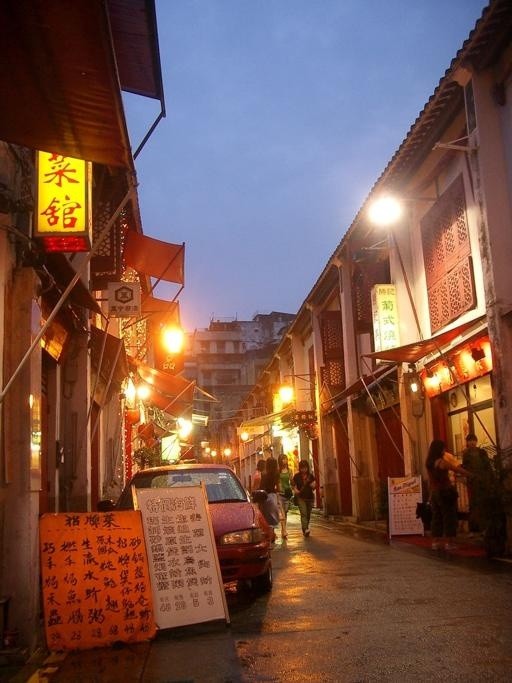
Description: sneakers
468,530,487,538
431,542,457,552
305,529,310,536
282,532,288,540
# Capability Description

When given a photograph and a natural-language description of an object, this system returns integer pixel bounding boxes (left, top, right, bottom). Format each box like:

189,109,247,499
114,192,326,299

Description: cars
98,464,273,596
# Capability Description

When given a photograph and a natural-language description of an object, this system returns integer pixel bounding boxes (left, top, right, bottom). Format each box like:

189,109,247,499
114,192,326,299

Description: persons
291,459,317,537
274,452,294,537
425,439,475,550
259,456,281,550
459,434,492,536
251,459,268,493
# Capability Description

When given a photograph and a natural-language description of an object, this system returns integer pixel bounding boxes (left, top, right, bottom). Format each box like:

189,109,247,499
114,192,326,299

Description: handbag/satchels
439,486,459,504
293,494,298,506
284,488,292,498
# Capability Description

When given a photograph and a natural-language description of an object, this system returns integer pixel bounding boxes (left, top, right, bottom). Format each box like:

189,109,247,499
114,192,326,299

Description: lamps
405,345,485,393
